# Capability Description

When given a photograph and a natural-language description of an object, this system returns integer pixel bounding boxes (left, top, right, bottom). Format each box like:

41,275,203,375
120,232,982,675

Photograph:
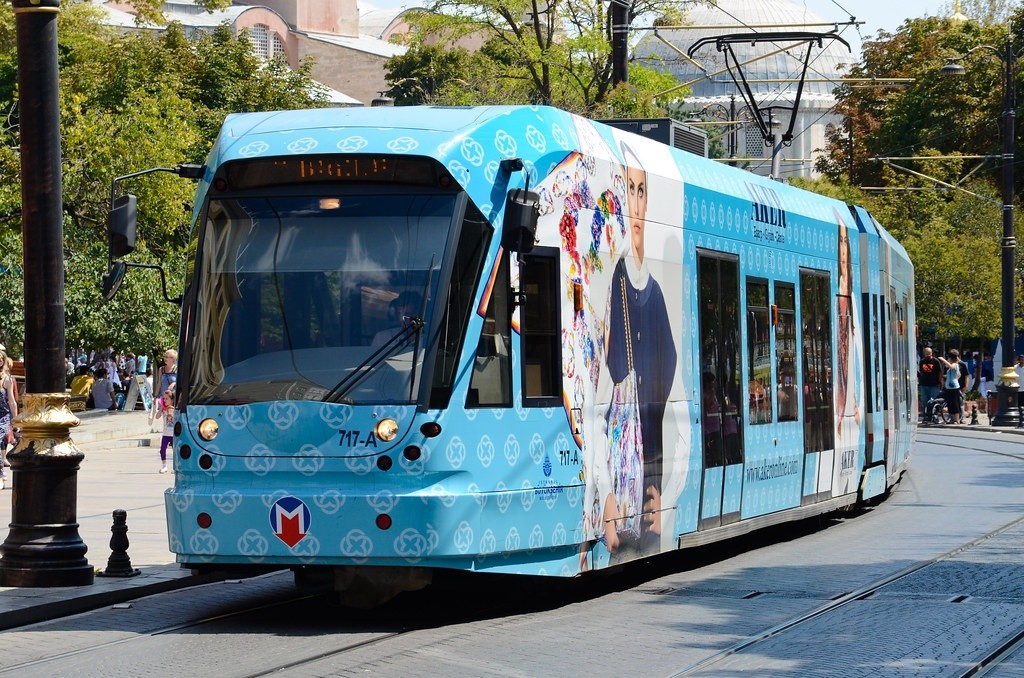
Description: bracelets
153,395,157,398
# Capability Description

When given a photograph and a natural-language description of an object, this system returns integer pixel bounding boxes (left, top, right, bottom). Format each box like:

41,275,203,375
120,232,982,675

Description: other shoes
159,467,168,473
0,483,5,490
947,420,960,425
922,418,928,424
4,459,11,467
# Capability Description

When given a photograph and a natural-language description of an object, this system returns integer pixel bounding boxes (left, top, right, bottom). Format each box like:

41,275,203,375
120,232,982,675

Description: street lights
939,34,1024,427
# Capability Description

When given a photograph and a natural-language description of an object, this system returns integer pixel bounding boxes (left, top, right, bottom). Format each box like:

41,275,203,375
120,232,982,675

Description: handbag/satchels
595,369,642,545
148,401,156,426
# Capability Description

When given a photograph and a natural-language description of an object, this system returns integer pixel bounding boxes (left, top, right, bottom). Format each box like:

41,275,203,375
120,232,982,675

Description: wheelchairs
925,390,964,424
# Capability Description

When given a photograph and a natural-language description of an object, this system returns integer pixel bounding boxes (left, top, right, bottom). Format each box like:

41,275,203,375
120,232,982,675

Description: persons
65,347,153,411
477,113,644,569
834,207,861,495
152,348,177,474
606,140,677,565
0,347,21,489
917,346,1024,425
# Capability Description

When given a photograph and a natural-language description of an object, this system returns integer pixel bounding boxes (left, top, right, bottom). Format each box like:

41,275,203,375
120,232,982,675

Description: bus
103,31,915,577
